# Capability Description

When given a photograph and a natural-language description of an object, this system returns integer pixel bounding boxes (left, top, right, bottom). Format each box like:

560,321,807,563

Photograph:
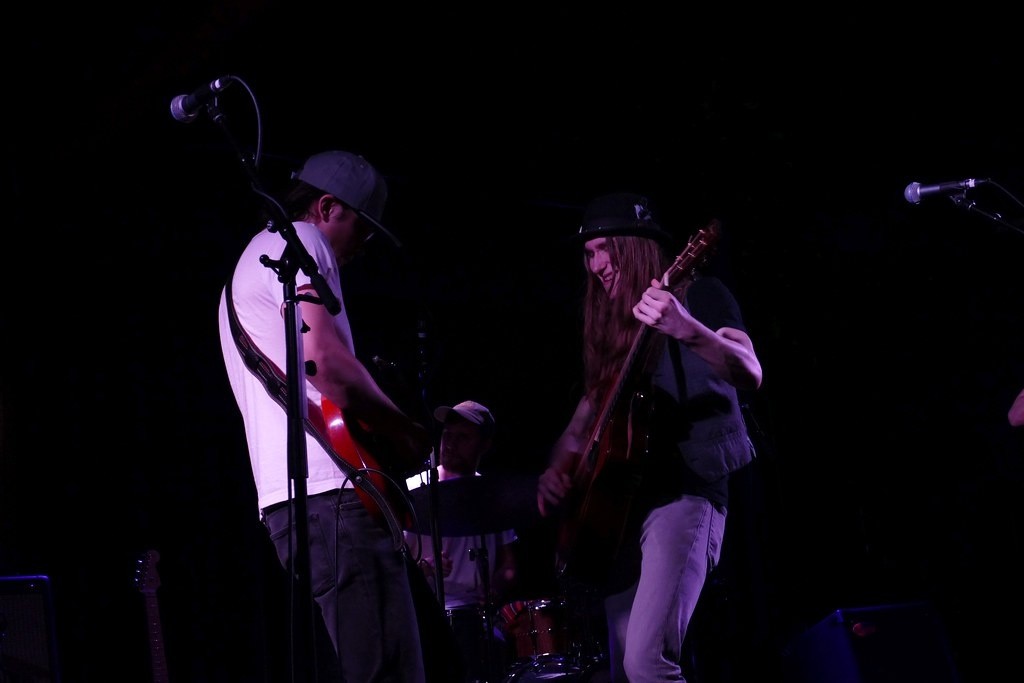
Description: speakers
0,574,62,682
784,602,960,683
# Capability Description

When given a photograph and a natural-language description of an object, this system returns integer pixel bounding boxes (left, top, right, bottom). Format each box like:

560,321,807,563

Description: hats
573,192,671,246
432,401,495,435
294,148,406,249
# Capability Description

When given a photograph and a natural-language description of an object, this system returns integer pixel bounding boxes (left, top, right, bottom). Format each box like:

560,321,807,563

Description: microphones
170,73,236,124
904,177,990,207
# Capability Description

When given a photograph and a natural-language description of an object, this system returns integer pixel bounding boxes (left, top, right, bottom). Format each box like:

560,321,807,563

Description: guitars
320,352,416,534
552,220,721,587
133,549,171,682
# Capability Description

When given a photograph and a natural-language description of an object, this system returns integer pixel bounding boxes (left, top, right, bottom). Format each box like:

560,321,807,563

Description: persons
405,401,522,649
535,197,765,683
216,150,435,683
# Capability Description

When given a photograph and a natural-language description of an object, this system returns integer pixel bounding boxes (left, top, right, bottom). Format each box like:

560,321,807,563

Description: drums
507,599,603,683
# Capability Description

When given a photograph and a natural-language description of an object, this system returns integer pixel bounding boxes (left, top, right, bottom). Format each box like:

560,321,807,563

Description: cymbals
394,468,542,538
